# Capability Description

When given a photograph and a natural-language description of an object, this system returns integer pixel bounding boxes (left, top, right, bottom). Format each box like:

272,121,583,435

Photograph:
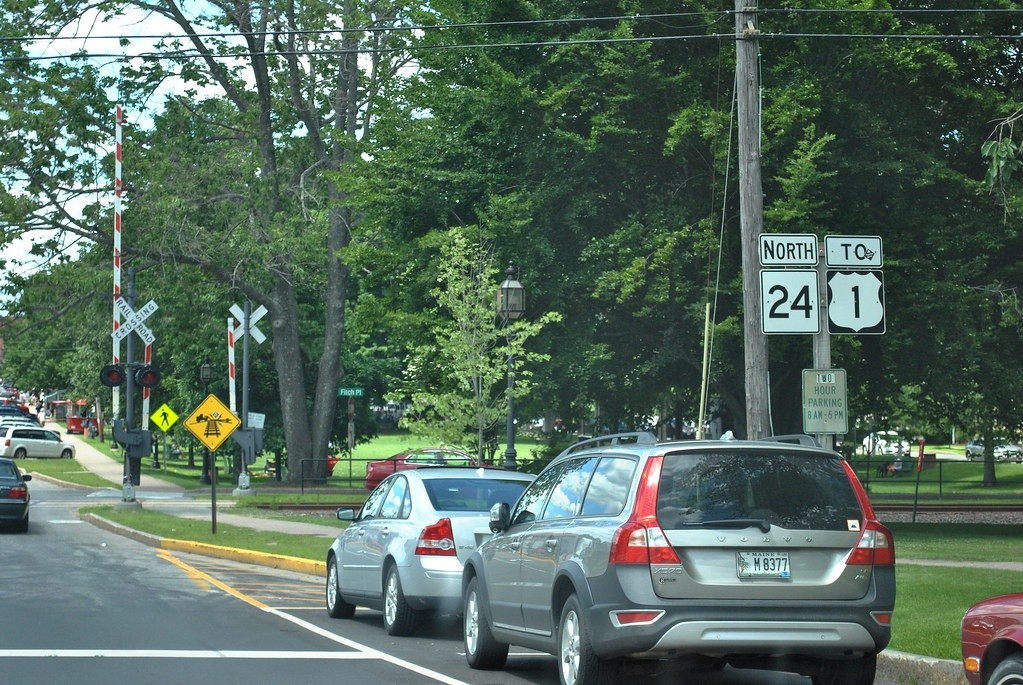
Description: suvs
457,429,899,685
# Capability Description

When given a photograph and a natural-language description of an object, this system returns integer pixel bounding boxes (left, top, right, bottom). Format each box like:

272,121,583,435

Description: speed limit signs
756,268,824,337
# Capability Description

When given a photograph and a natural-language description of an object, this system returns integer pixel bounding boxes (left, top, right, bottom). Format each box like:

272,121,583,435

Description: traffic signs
338,387,364,398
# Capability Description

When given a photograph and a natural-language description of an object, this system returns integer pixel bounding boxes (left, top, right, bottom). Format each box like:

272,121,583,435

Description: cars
0,378,105,460
0,457,32,533
322,464,540,636
959,592,1023,685
864,429,911,456
964,435,1023,464
363,448,509,493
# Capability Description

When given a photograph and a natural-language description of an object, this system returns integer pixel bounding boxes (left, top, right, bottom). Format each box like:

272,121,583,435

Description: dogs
877,463,890,477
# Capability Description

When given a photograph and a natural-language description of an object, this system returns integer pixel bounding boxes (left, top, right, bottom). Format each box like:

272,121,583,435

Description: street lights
495,259,527,472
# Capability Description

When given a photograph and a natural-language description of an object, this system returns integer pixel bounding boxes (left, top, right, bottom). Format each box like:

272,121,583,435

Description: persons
889,458,903,477
12,388,46,427
88,403,96,417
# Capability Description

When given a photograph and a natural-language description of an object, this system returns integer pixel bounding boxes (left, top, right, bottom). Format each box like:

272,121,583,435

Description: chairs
660,468,749,514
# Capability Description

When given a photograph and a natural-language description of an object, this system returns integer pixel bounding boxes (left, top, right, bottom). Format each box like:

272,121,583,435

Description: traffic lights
134,364,162,389
98,364,127,387
